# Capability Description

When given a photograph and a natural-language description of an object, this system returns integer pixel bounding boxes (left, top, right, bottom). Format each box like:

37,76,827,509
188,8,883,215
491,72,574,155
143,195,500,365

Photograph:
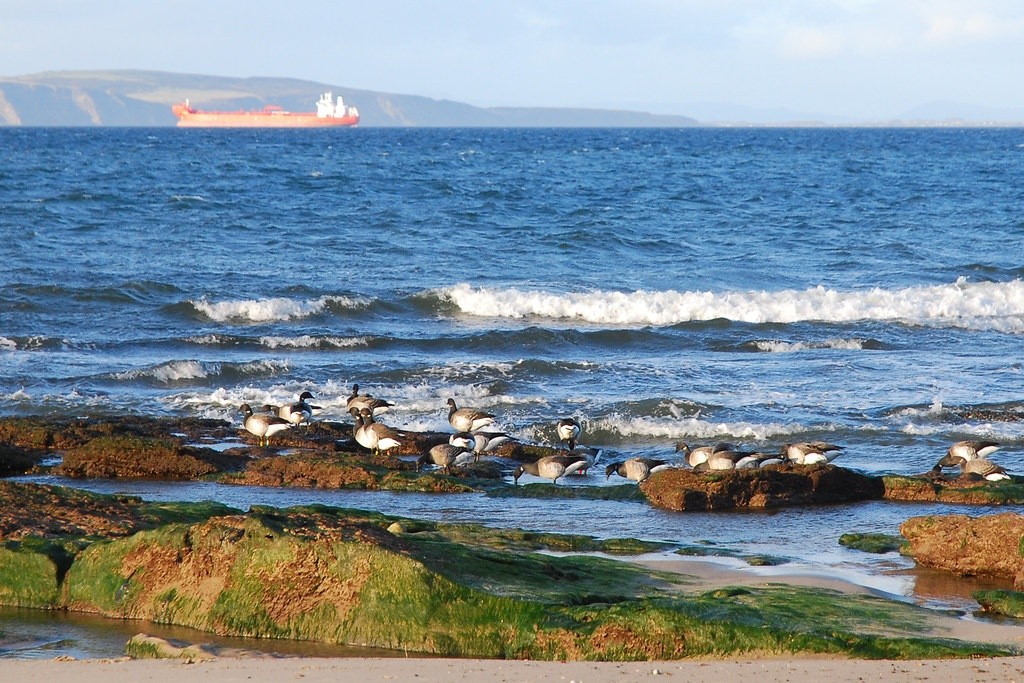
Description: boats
172,89,360,128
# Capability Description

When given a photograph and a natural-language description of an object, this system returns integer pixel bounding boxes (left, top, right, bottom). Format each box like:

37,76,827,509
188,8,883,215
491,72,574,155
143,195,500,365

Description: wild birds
346,407,411,457
675,440,784,470
557,418,581,451
416,429,520,475
447,397,497,435
513,448,603,487
236,404,292,447
932,439,1001,477
347,383,394,423
605,457,669,483
947,455,1012,482
262,392,323,433
779,442,847,468
